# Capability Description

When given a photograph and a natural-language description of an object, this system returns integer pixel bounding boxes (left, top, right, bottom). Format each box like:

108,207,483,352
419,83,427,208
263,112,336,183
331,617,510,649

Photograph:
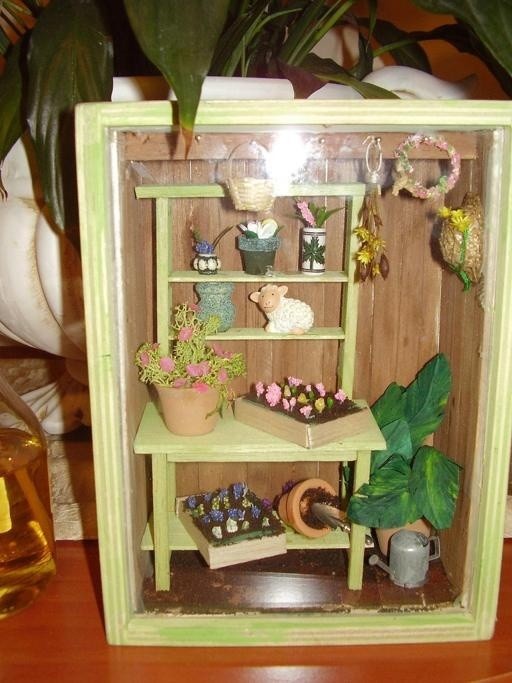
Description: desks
130,395,388,595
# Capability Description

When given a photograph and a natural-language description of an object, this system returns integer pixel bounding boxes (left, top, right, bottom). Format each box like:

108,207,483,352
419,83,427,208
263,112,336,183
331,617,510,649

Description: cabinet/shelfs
126,178,388,557
71,95,512,651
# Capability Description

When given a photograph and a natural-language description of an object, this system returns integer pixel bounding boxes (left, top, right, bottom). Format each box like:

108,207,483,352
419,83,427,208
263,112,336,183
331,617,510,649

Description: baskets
225,141,278,211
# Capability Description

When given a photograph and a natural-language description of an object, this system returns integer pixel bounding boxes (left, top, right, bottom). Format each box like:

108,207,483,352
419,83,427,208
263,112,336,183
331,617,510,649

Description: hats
439,192,484,292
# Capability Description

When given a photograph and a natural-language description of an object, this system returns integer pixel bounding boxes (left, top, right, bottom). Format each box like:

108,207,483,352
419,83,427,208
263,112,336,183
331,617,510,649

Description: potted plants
335,346,466,560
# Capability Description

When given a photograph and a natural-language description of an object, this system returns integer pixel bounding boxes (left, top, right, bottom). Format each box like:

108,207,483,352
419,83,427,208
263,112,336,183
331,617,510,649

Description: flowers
388,130,463,202
249,370,350,421
350,134,390,283
434,203,475,295
276,188,350,229
131,294,248,421
233,214,286,240
181,475,283,545
188,222,236,255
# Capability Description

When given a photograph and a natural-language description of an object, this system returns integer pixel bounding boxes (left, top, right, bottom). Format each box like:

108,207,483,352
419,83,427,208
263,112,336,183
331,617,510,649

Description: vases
232,232,282,277
276,476,338,539
298,223,328,277
144,373,224,437
190,252,223,275
